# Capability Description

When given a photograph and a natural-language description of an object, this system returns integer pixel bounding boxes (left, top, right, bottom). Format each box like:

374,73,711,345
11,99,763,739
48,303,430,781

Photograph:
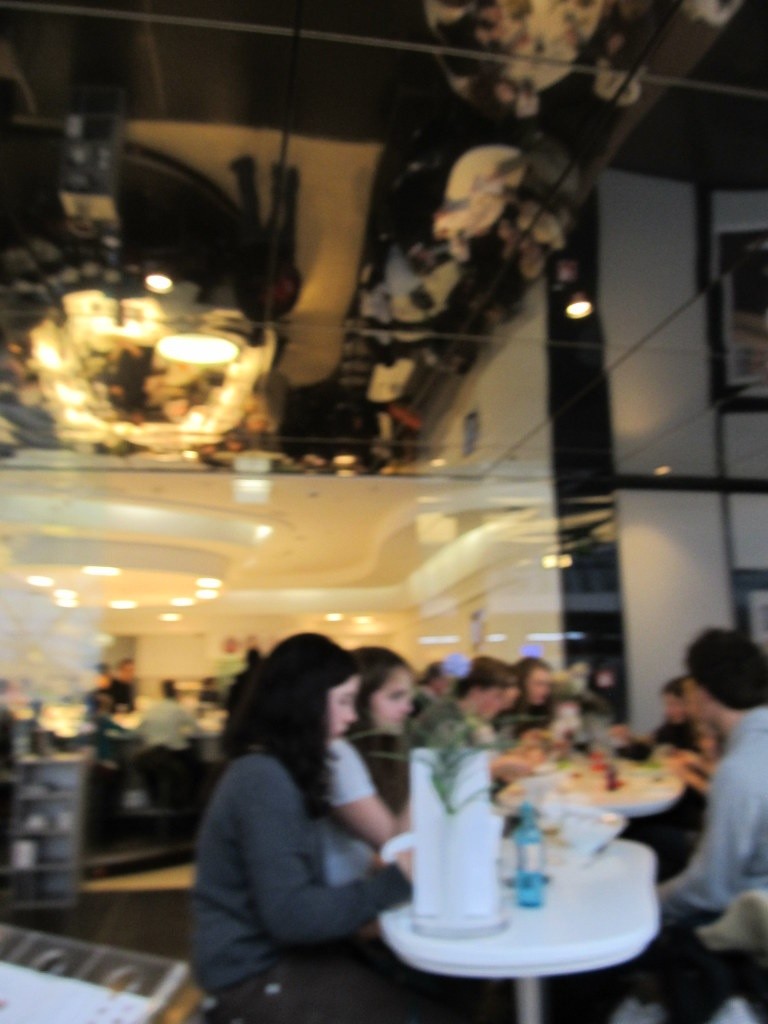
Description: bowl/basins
543,811,626,854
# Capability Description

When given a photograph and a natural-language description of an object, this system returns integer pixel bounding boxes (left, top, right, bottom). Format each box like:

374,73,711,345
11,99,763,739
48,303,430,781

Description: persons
338,0,617,465
0,626,768,1024
324,644,413,891
190,633,410,1024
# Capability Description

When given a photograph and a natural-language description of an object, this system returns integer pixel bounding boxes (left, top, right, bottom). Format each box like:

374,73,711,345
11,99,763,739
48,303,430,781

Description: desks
378,762,687,1023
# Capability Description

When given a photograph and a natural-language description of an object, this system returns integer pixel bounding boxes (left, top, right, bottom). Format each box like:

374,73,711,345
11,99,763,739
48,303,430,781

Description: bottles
513,802,543,910
